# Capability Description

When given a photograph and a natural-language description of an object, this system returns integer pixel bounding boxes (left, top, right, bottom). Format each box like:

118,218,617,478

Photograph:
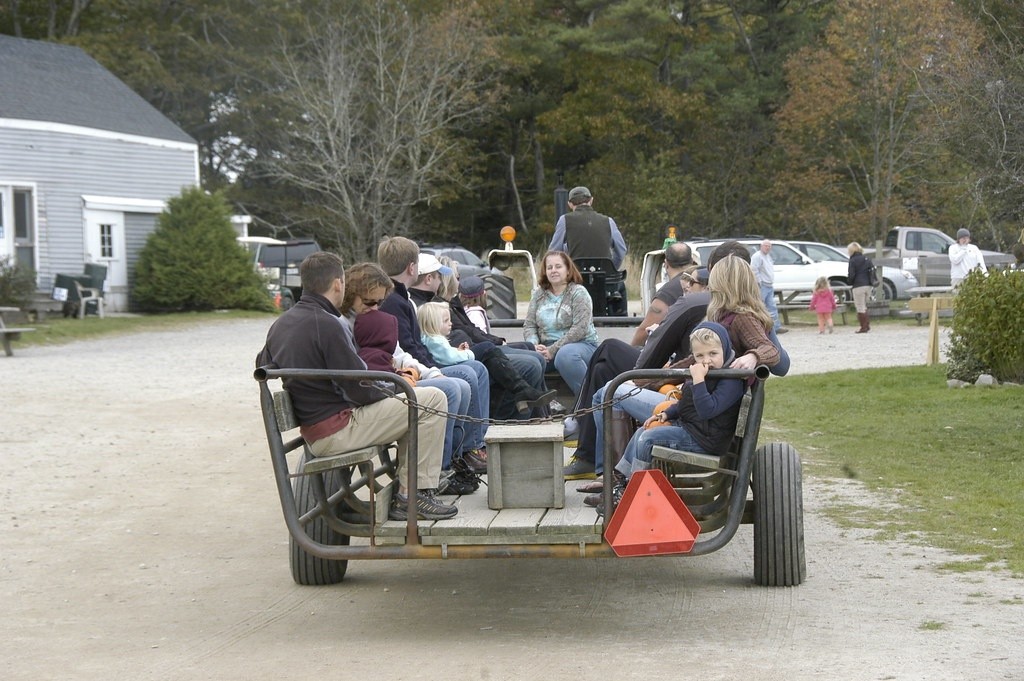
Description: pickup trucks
827,225,1016,294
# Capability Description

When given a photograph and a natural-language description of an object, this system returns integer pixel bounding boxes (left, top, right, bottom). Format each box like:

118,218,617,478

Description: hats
957,228,970,239
568,186,592,200
458,276,484,297
417,251,452,275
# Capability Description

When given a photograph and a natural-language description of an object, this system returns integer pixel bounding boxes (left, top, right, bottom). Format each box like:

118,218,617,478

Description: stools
484,425,566,511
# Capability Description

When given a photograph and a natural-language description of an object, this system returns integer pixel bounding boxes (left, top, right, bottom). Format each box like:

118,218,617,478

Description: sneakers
563,454,598,480
421,470,479,496
388,491,458,520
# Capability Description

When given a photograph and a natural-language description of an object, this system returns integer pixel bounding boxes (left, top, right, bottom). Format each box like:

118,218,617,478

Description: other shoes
577,473,619,492
597,486,630,514
776,328,788,334
820,326,834,335
463,451,487,474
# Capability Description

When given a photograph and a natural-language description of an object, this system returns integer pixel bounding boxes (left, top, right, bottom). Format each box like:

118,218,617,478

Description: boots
483,349,559,415
855,311,870,333
584,410,636,506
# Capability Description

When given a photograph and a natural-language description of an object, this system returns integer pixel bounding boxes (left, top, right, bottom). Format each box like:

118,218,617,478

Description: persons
846,242,879,333
948,228,989,291
750,239,788,334
255,187,790,521
809,277,837,334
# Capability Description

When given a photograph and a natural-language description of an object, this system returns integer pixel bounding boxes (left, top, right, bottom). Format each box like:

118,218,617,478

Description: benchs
253,363,393,536
774,298,846,324
644,362,771,521
0,328,37,356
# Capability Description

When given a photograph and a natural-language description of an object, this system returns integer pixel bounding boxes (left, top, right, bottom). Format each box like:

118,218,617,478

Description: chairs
75,280,105,319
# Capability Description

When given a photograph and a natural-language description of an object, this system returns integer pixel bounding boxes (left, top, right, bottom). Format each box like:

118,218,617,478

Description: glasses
690,279,702,287
358,294,385,307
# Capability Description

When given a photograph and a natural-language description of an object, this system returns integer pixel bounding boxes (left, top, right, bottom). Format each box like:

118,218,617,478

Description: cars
238,236,322,307
642,241,917,321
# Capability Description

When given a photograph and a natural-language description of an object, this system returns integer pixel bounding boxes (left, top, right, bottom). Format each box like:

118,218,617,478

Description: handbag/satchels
865,257,880,287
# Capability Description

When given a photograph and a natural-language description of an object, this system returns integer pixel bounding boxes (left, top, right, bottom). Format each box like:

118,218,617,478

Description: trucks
256,224,807,592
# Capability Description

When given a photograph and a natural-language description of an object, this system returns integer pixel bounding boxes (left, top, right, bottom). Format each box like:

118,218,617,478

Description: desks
1,306,21,355
906,286,955,321
771,286,850,324
